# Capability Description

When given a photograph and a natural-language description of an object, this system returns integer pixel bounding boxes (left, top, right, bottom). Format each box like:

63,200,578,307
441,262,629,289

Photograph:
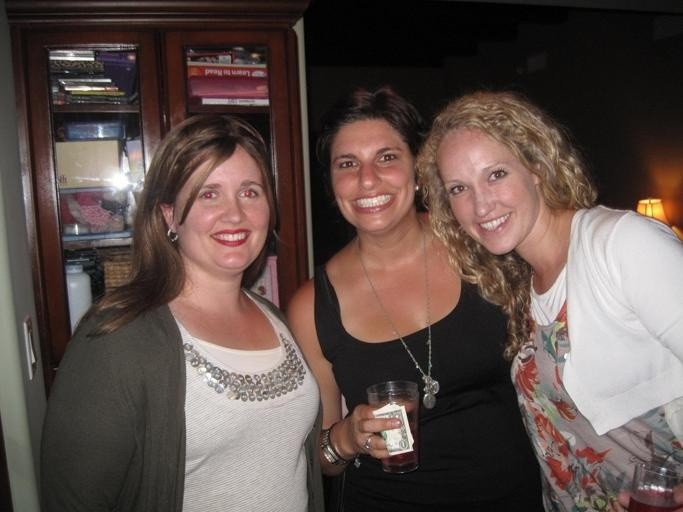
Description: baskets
102,252,135,296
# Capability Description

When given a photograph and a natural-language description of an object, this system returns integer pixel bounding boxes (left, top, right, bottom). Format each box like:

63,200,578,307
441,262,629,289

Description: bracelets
322,421,350,472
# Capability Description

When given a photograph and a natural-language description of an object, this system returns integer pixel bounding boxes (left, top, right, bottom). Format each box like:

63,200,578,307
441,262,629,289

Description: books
55,122,144,289
52,48,139,105
186,47,271,107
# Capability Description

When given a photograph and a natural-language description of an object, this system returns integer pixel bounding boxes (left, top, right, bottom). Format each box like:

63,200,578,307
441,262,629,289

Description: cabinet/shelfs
1,1,312,402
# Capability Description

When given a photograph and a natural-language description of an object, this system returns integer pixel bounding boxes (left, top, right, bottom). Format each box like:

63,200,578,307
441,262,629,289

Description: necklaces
347,216,441,410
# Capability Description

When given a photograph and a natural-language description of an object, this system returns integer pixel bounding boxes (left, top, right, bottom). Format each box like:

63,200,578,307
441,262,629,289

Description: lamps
633,196,670,230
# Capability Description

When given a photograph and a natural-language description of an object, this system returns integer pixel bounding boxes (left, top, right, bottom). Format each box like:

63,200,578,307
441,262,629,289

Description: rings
362,434,375,452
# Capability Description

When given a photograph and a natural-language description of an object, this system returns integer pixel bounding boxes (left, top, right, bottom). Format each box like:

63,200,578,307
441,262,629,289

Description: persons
287,86,545,510
38,112,321,510
415,89,681,512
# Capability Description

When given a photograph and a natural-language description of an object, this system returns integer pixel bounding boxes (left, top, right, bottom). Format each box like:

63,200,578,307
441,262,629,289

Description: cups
365,378,420,477
627,461,682,512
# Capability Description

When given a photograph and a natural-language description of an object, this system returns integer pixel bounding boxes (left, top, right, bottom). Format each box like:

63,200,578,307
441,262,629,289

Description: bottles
65,263,93,335
